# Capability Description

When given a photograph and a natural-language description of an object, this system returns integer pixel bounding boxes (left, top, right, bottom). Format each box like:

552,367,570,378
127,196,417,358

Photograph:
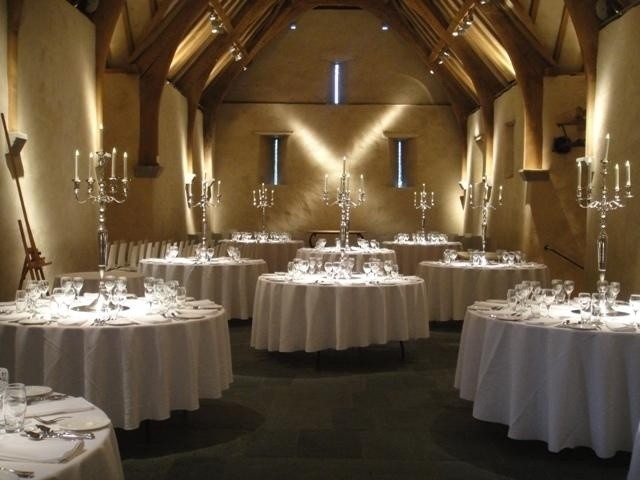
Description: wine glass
467,248,486,266
629,294,640,317
116,276,128,294
564,280,574,306
25,279,49,311
63,288,75,315
319,238,327,250
143,276,186,310
578,293,590,317
506,280,541,313
392,231,449,244
333,262,341,276
114,287,127,317
324,262,333,277
610,282,620,308
231,231,289,242
356,237,380,250
551,279,563,305
596,280,608,302
60,277,72,294
363,257,398,281
542,289,555,315
170,246,179,260
207,248,214,263
287,257,322,277
99,289,112,315
495,249,522,266
105,275,116,302
442,248,457,265
53,288,65,315
72,276,83,302
227,246,241,262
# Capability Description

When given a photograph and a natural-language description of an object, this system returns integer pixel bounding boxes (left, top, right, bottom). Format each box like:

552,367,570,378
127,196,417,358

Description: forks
33,416,72,425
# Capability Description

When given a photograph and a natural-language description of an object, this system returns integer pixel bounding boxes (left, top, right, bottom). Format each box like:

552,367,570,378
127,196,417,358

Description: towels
606,318,637,332
24,396,95,419
0,436,86,462
57,315,91,325
136,314,172,324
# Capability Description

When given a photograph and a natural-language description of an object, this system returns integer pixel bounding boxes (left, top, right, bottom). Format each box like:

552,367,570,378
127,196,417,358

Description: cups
4,382,27,433
16,290,25,312
591,293,605,318
0,368,8,410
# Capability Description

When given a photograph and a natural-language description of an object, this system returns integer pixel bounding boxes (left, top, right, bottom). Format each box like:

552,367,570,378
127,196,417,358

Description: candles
360,178,363,192
614,167,619,190
111,152,116,178
625,164,631,186
603,138,609,162
577,166,582,188
75,154,79,179
342,160,345,175
123,157,127,178
217,183,220,195
88,157,92,178
324,177,328,192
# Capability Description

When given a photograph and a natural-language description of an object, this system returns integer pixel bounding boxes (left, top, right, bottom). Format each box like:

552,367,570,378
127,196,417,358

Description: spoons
25,430,96,441
36,424,94,436
557,320,570,326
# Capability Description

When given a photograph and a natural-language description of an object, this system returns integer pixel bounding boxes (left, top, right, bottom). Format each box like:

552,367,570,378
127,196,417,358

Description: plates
177,313,204,319
568,323,598,330
106,320,132,326
52,417,111,430
5,385,53,398
19,319,47,325
491,313,522,321
